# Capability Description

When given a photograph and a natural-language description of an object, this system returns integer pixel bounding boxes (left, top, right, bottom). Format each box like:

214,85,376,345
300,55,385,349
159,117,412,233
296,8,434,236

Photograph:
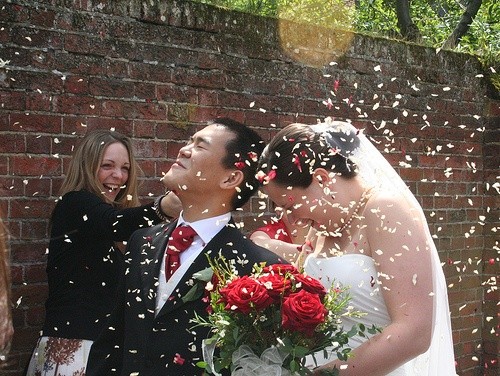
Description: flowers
189,248,383,376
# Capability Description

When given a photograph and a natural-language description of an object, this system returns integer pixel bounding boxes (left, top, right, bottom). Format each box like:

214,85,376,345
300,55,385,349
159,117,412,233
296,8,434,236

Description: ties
165,224,198,282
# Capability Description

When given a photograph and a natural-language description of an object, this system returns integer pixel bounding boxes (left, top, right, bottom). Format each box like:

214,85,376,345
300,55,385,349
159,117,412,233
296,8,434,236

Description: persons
250,204,310,263
119,118,291,376
42,129,183,376
256,121,457,376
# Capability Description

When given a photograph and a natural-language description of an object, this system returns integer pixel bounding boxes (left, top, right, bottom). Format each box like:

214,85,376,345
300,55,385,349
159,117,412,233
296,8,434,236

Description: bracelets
154,194,169,221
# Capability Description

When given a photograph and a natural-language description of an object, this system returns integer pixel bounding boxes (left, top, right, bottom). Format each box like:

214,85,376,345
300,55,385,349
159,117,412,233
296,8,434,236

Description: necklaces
339,184,376,233
